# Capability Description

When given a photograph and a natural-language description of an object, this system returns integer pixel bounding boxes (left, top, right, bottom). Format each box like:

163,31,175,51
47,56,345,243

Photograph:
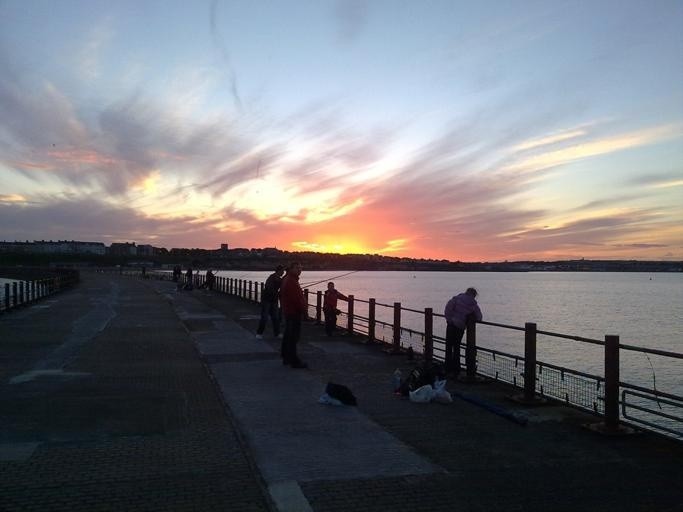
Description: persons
443,287,483,372
255,260,308,369
141,264,145,276
323,281,354,336
172,265,216,291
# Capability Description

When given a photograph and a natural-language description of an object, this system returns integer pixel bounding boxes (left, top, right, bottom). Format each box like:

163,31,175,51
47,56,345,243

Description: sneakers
255,334,263,339
282,357,288,365
290,359,307,368
272,333,283,339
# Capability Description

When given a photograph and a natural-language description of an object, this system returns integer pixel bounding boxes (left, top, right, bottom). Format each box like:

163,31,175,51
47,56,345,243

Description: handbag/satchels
427,361,447,384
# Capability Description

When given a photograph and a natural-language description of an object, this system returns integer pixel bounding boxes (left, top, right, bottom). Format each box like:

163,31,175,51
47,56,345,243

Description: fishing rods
301,270,357,290
298,280,320,285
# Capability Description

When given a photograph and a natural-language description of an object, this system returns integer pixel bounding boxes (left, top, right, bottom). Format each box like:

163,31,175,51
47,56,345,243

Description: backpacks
396,367,435,398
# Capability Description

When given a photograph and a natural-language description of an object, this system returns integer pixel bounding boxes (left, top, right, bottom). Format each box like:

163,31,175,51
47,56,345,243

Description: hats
276,265,287,271
